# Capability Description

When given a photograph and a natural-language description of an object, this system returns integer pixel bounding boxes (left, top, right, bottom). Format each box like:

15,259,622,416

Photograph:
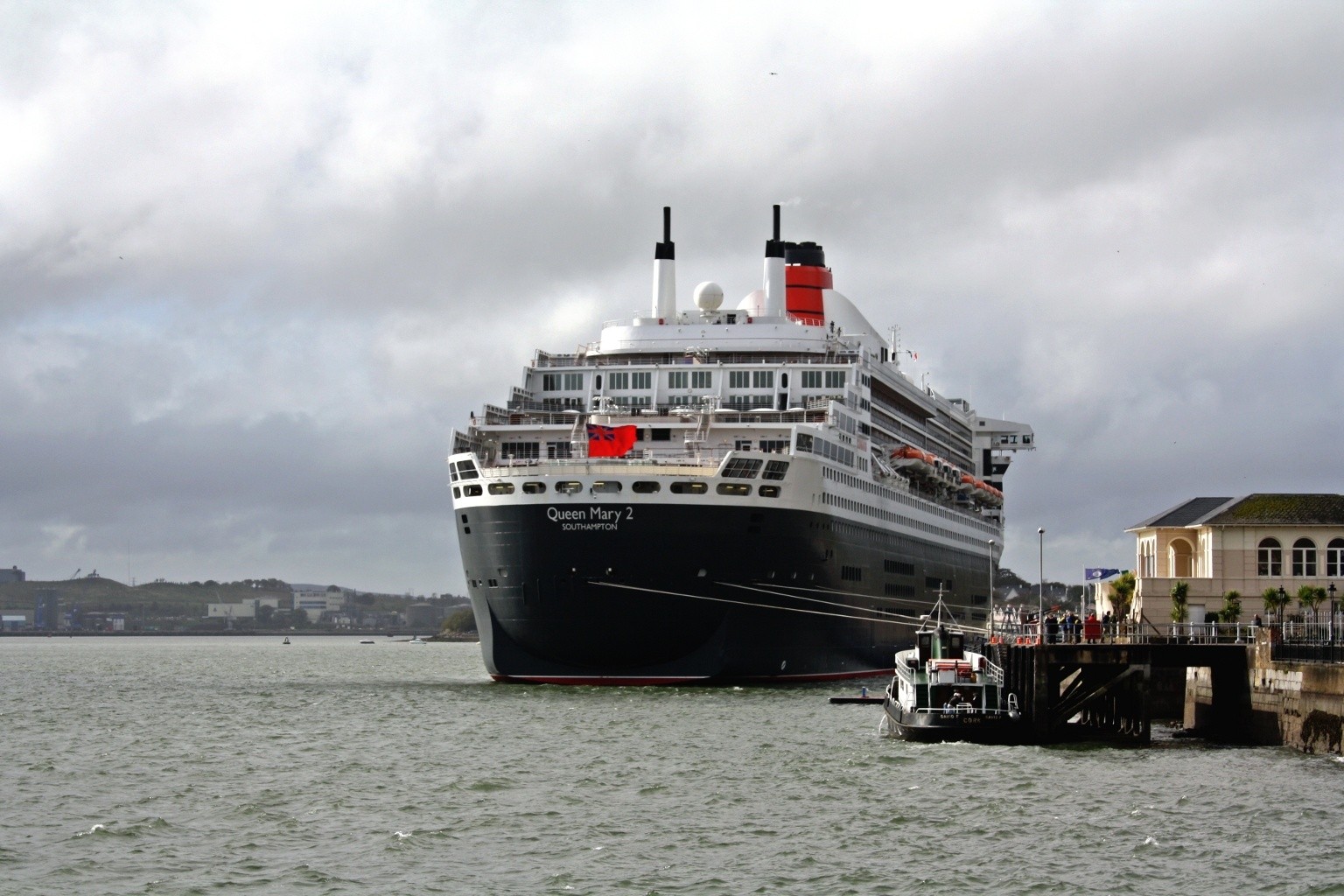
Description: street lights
988,539,996,641
1037,527,1045,644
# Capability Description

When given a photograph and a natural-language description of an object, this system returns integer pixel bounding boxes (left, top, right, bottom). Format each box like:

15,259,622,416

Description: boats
283,636,291,644
899,445,1006,504
409,638,427,645
880,581,1021,745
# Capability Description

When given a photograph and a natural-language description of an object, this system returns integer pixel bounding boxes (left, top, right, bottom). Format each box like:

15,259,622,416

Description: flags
585,422,638,459
1085,568,1121,580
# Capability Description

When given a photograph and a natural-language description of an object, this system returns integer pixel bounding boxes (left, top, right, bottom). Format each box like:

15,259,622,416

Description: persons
1253,613,1265,628
1044,608,1116,644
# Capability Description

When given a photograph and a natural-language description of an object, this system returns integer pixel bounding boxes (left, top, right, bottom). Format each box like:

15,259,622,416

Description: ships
446,204,1040,690
